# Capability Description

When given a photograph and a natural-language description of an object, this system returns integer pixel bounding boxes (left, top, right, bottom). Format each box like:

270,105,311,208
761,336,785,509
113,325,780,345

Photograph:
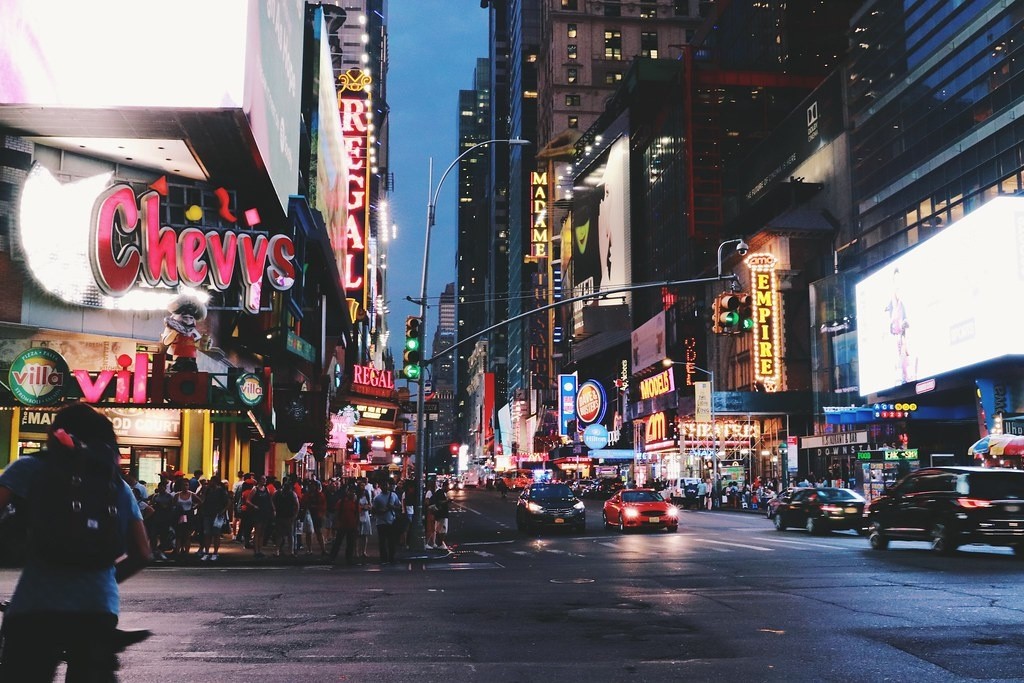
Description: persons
726,475,777,508
232,471,326,558
626,477,638,489
374,478,453,565
883,268,911,384
322,476,375,561
411,473,415,480
798,476,828,487
0,403,154,683
123,469,234,561
643,478,661,491
163,295,207,372
696,478,712,510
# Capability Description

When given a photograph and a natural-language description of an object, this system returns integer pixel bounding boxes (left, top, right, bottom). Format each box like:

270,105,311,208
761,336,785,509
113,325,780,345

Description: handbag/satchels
213,513,224,528
395,516,400,537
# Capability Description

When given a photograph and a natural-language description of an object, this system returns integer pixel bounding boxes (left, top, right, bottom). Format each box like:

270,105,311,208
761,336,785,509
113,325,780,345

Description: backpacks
24,458,124,568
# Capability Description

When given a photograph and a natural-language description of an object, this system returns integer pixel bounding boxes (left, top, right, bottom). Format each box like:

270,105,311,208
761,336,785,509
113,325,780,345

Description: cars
443,479,464,493
516,483,586,536
766,487,806,519
773,487,868,536
669,478,702,499
565,477,626,501
603,488,679,534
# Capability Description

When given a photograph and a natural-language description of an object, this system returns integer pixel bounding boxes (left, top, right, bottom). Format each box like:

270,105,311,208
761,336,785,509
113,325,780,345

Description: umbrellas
968,434,1015,456
990,435,1024,455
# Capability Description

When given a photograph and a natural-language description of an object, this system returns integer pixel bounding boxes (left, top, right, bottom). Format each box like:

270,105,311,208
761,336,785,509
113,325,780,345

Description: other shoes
440,541,448,549
425,544,433,549
210,555,219,560
200,554,210,560
431,544,437,548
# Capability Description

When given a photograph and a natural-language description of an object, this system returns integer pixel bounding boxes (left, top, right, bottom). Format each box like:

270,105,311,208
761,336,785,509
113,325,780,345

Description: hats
174,470,186,476
245,478,256,484
158,471,169,477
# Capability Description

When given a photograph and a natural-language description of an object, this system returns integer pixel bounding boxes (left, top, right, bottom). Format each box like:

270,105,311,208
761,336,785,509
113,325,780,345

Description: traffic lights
450,443,459,453
403,349,419,379
406,317,419,350
497,444,504,455
383,434,401,453
716,292,741,328
738,293,754,332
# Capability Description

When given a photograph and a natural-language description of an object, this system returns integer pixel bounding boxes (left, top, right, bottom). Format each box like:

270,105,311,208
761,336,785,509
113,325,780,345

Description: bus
501,468,534,492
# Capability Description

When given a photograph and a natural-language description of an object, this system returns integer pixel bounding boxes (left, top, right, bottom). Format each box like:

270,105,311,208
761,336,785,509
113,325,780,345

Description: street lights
662,359,718,494
411,137,534,554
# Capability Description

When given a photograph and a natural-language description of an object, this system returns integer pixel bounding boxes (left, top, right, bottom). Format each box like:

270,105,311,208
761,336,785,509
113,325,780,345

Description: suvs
867,466,1024,558
